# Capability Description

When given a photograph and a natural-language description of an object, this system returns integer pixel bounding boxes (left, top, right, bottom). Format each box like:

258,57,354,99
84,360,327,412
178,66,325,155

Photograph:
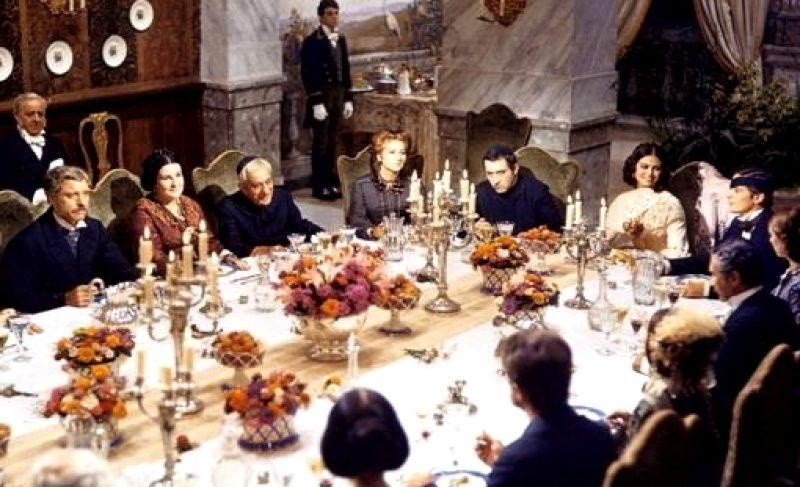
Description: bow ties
23,135,47,147
731,217,757,233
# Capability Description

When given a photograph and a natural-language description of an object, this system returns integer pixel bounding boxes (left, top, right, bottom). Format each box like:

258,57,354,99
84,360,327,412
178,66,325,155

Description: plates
571,405,606,422
0,47,14,83
102,34,128,67
45,40,74,76
129,0,155,32
430,469,489,487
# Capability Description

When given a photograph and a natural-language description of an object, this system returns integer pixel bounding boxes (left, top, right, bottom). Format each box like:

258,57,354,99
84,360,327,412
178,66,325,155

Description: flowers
219,373,310,423
732,62,797,143
276,256,371,317
369,274,422,309
45,368,124,421
212,330,261,353
469,234,528,270
517,224,565,254
498,272,561,314
330,240,389,282
56,324,134,365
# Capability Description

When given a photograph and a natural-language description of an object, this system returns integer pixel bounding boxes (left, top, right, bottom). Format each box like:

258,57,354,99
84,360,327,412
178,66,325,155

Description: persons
318,387,438,486
0,91,65,203
125,149,251,275
346,130,426,240
704,240,800,436
767,190,800,323
605,141,691,262
607,303,726,456
648,168,790,301
299,0,354,201
211,154,326,258
1,164,141,313
474,326,619,486
456,145,567,237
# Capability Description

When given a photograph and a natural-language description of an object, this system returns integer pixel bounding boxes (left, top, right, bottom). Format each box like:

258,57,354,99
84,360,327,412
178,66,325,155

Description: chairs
337,145,373,221
91,168,146,230
667,160,739,256
606,406,699,487
719,346,800,486
0,189,40,246
193,149,259,195
494,145,580,203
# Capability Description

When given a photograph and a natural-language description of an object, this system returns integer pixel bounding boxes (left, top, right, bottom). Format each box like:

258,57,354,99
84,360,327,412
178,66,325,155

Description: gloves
313,104,328,120
343,101,354,119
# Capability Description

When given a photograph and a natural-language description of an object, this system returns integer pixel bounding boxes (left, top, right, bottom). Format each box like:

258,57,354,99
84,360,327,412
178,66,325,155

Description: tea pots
61,411,111,459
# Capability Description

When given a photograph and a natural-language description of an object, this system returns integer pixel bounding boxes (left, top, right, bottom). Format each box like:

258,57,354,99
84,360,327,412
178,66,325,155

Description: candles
206,253,220,305
139,225,154,264
142,265,155,304
80,0,85,8
468,183,476,213
599,197,607,229
136,350,143,378
499,0,505,16
69,0,74,11
184,345,192,369
166,250,176,284
198,219,207,264
159,365,172,388
409,169,420,198
182,228,194,277
574,189,582,221
432,169,442,220
460,168,469,200
442,158,451,191
565,194,573,228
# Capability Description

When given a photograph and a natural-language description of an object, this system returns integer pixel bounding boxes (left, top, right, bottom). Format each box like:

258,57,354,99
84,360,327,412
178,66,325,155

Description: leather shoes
329,187,342,198
312,184,334,200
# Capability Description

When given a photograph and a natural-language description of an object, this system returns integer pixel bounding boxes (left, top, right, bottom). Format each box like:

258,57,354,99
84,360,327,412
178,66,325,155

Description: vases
299,314,368,362
70,420,125,451
374,298,419,334
236,412,299,448
480,266,518,295
217,354,262,386
528,251,554,274
514,308,544,331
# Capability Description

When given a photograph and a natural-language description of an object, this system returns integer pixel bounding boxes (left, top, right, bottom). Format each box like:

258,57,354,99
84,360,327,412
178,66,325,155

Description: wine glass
10,316,30,352
595,311,617,355
497,220,515,237
287,232,306,253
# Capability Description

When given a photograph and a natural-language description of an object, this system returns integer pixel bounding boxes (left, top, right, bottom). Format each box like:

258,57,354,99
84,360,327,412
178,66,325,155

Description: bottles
396,62,411,95
210,428,251,487
